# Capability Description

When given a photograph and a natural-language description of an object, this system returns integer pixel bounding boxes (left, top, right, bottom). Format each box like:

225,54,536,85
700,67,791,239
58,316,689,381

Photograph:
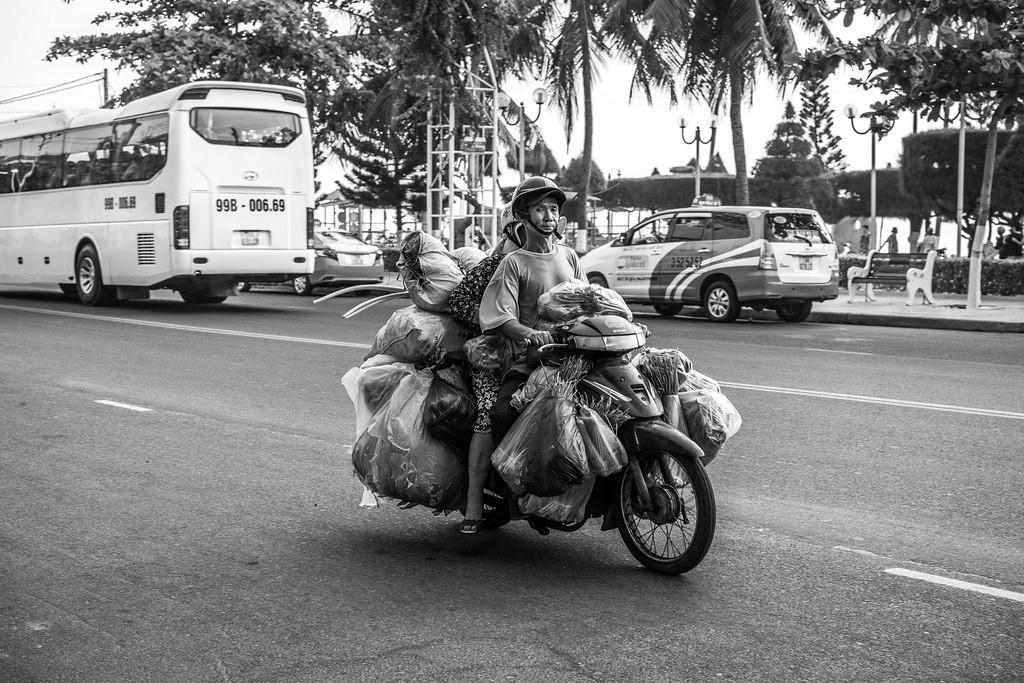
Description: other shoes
508,494,529,519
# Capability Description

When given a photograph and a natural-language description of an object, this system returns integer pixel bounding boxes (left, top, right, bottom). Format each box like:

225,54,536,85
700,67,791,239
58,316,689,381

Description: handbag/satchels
340,278,740,525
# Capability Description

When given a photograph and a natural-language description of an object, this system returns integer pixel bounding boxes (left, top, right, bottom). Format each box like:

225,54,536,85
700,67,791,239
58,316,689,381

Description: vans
580,206,840,323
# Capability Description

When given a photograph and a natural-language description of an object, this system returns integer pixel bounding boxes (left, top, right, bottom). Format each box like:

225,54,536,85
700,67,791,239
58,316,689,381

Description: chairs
45,152,161,190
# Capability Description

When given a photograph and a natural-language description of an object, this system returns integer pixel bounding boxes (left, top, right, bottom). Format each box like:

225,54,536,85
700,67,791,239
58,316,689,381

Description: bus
0,81,316,305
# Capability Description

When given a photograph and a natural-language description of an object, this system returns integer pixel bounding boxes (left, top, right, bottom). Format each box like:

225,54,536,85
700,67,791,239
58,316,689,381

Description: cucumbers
576,412,628,477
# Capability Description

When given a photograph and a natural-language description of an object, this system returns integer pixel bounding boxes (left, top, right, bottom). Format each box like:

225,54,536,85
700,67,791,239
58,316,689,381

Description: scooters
460,315,717,574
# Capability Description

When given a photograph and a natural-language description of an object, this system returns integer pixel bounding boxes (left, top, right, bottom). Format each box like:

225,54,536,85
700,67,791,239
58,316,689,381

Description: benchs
846,249,938,308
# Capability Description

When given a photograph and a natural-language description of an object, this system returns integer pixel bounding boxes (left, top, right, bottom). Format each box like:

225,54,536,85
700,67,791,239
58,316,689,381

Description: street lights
680,116,718,196
845,104,895,253
497,88,548,180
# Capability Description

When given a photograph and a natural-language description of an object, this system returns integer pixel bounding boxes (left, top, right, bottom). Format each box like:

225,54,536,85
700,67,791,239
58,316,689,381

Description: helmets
501,201,521,234
511,176,567,219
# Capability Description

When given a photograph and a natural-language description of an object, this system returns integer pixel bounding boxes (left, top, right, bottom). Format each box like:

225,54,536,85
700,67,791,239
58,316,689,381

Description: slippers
460,518,483,534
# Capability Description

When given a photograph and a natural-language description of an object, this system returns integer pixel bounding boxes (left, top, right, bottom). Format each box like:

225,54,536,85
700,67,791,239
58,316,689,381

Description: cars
238,228,384,296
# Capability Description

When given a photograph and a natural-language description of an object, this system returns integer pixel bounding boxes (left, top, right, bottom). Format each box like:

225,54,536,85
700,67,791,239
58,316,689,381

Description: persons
480,176,645,519
878,227,898,253
961,224,974,257
858,225,870,253
469,217,486,251
919,228,936,251
994,223,1024,259
446,202,523,532
